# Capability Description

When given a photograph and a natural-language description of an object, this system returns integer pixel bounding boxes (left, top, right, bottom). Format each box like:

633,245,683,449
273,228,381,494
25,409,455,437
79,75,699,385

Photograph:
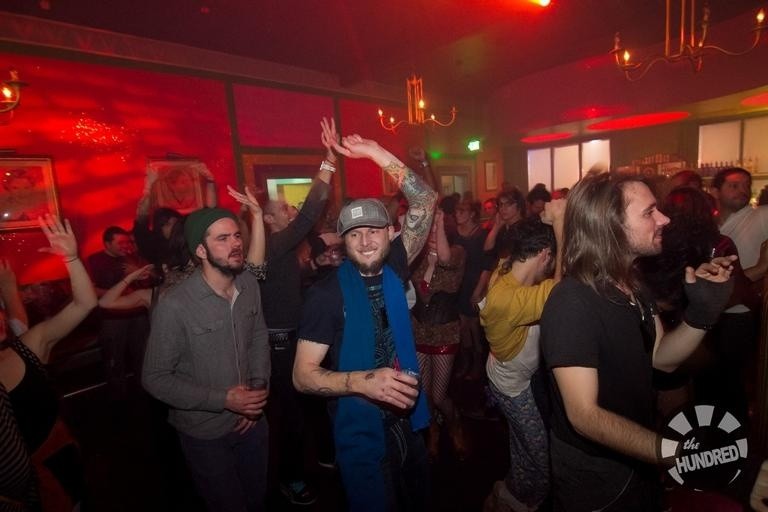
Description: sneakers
279,478,318,506
461,405,501,422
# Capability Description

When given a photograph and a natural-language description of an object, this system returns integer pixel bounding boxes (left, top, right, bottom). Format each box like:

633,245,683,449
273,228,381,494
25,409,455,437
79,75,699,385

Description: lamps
0,69,31,113
608,0,768,82
378,75,458,132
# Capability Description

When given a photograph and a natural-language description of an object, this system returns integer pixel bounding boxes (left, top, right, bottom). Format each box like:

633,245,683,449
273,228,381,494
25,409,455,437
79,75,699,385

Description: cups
244,368,268,391
398,367,423,411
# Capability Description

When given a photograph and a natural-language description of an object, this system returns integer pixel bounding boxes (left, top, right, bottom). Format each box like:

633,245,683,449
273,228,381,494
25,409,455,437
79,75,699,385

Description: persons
0,115,768,510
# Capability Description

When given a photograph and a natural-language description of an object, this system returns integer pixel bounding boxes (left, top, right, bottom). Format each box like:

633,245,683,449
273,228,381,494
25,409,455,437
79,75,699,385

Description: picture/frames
484,161,499,191
381,168,399,196
147,158,204,216
0,155,61,232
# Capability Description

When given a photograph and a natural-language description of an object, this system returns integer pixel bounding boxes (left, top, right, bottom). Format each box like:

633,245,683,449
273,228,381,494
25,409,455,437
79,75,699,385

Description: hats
182,207,239,257
336,198,390,239
